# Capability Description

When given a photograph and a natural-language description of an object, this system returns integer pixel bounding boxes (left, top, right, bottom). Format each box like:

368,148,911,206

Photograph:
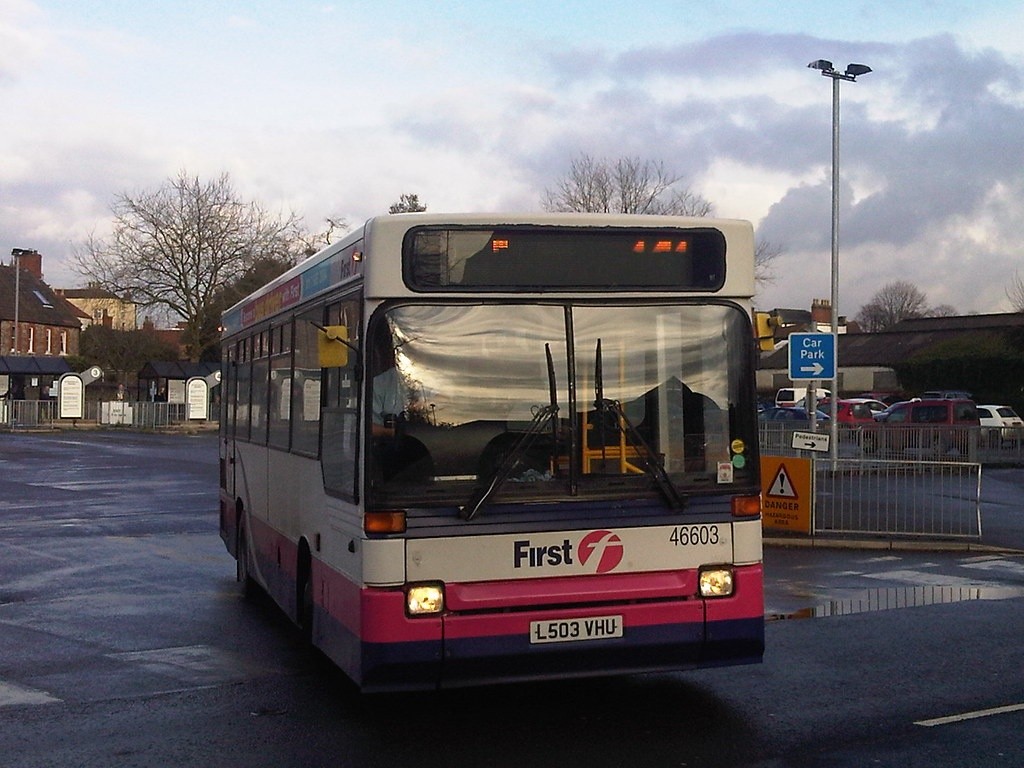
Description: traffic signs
791,430,831,453
788,332,837,380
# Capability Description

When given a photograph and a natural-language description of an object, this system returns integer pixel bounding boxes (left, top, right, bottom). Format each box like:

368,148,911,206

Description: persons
41,385,50,422
815,388,846,414
369,350,430,436
213,394,220,421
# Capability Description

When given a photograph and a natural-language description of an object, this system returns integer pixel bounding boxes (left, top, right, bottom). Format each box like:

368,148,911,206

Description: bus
221,210,783,694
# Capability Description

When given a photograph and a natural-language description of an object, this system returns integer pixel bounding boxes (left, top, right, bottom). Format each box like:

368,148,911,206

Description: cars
758,386,1024,459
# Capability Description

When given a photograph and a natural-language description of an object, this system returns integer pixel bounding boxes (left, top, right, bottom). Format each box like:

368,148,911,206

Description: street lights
430,403,437,427
10,251,24,357
807,60,875,476
